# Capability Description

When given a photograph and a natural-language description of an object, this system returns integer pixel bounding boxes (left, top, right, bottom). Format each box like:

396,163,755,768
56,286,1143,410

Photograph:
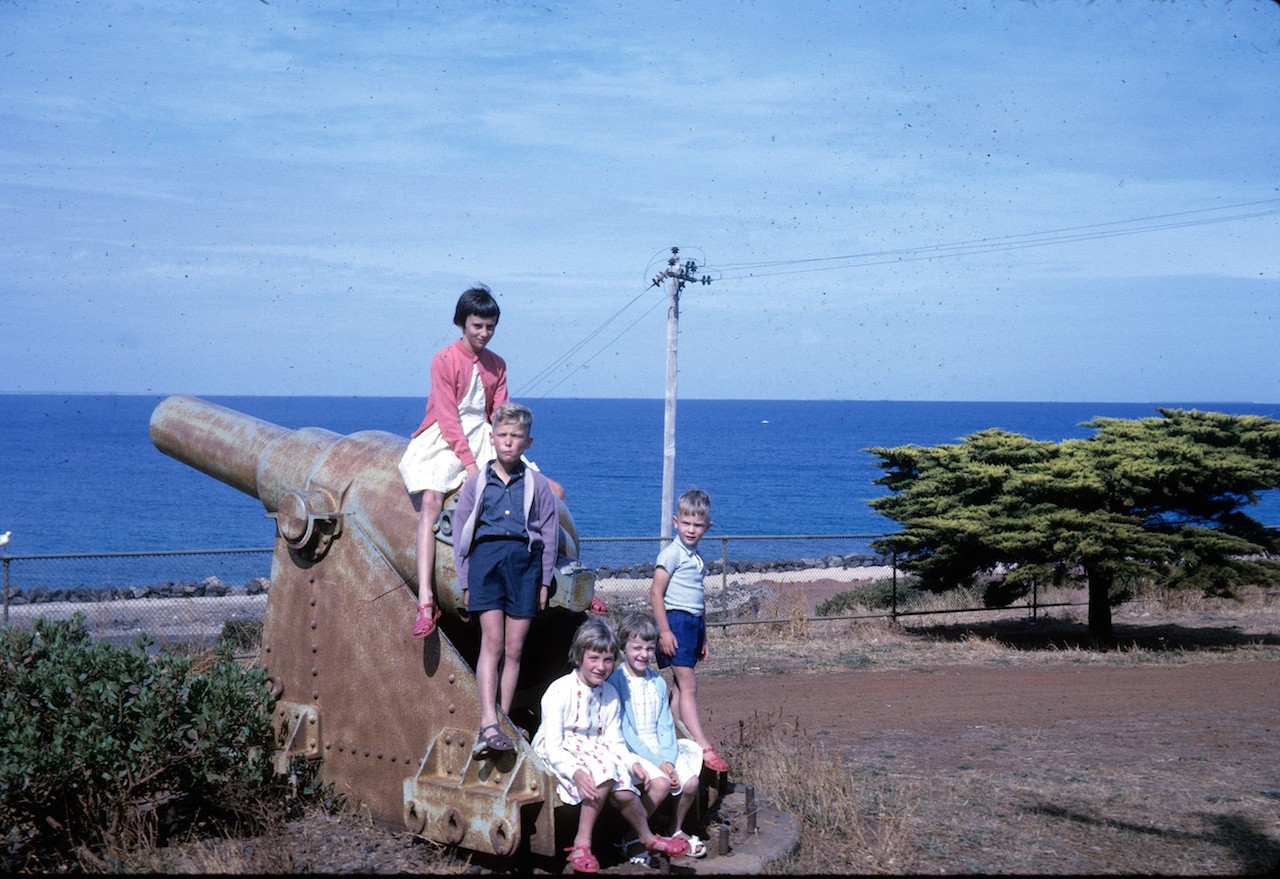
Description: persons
531,489,730,873
400,284,567,641
454,403,558,761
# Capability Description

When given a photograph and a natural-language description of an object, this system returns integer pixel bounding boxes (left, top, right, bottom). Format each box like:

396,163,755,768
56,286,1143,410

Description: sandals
412,602,441,639
587,595,609,615
671,830,706,857
703,748,730,771
564,845,599,872
647,833,691,857
478,725,515,749
